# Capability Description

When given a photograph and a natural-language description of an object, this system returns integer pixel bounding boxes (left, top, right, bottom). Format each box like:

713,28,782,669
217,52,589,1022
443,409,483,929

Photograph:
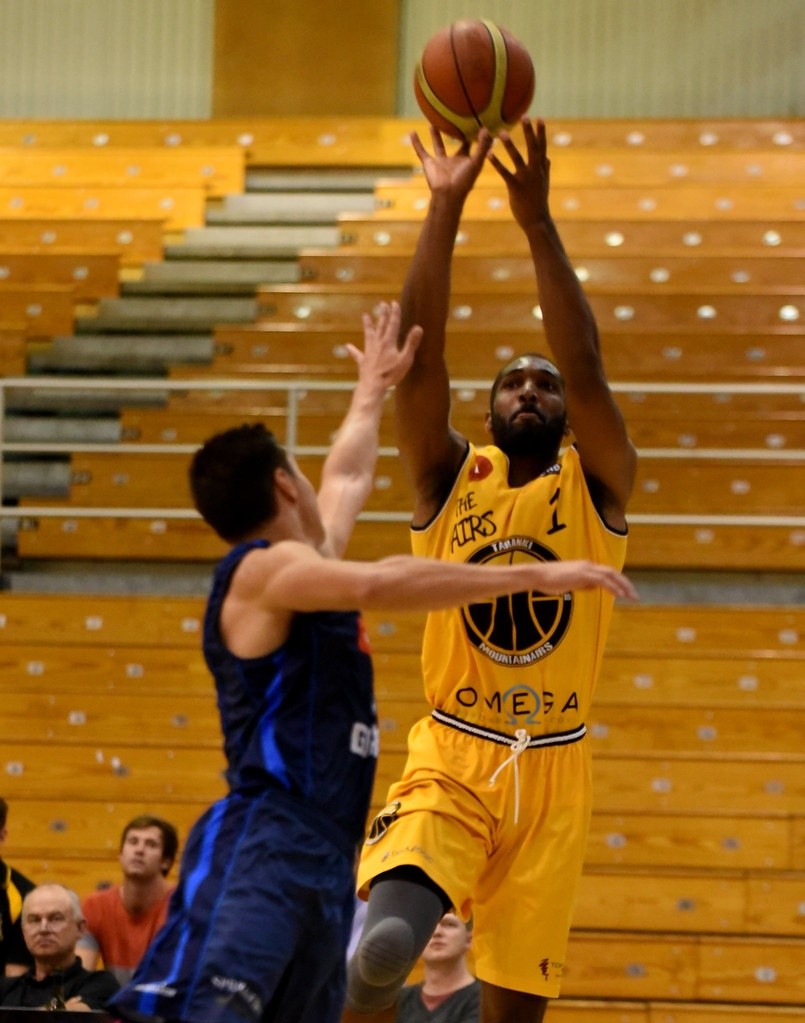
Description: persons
342,116,641,1022
94,299,639,1023
0,796,37,979
397,913,480,1023
0,885,121,1012
74,816,179,985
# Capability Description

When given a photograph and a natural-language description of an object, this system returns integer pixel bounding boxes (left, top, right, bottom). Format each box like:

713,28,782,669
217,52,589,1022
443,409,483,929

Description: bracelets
48,997,66,1011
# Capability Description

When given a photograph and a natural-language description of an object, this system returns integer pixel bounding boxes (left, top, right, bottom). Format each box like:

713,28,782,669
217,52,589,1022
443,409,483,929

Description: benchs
0,121,805,1023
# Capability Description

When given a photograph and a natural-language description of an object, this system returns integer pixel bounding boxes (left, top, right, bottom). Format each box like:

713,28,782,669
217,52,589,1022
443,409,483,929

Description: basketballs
413,16,537,145
